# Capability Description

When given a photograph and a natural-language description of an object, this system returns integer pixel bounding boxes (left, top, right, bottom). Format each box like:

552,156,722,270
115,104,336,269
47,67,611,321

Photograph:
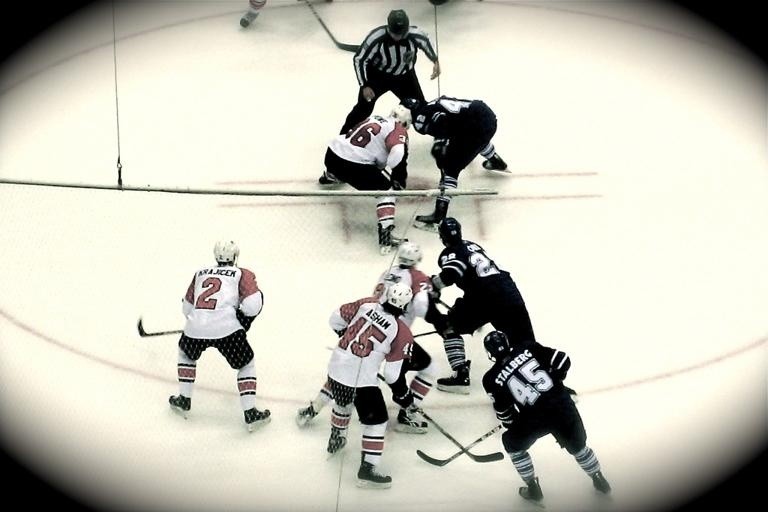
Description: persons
168,239,273,432
403,94,513,231
239,0,267,29
339,9,441,137
320,105,412,255
294,242,434,433
435,215,536,394
482,331,614,509
324,283,414,488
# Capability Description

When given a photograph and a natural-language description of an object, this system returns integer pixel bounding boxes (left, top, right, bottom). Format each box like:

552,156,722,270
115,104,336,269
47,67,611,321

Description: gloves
386,381,415,408
431,275,446,291
237,310,254,329
392,166,406,191
436,309,447,330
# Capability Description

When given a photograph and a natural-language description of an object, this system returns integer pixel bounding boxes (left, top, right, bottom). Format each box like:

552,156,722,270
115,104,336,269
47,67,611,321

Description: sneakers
242,11,258,25
592,475,604,488
328,429,343,454
320,166,345,184
397,412,429,428
376,224,407,245
483,153,507,171
301,402,316,422
245,407,269,424
438,363,471,386
359,454,389,483
391,105,412,130
416,199,448,222
520,480,542,500
403,97,420,109
169,397,189,410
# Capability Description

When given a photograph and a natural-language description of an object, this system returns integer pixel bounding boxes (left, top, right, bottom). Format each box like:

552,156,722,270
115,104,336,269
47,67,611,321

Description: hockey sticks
416,424,503,467
412,403,504,463
306,0,361,53
137,316,184,337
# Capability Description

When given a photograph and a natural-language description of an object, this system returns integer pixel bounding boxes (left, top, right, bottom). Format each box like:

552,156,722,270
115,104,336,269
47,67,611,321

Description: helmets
439,218,462,245
214,240,240,262
398,241,423,267
485,331,508,359
387,283,412,309
389,9,409,36
